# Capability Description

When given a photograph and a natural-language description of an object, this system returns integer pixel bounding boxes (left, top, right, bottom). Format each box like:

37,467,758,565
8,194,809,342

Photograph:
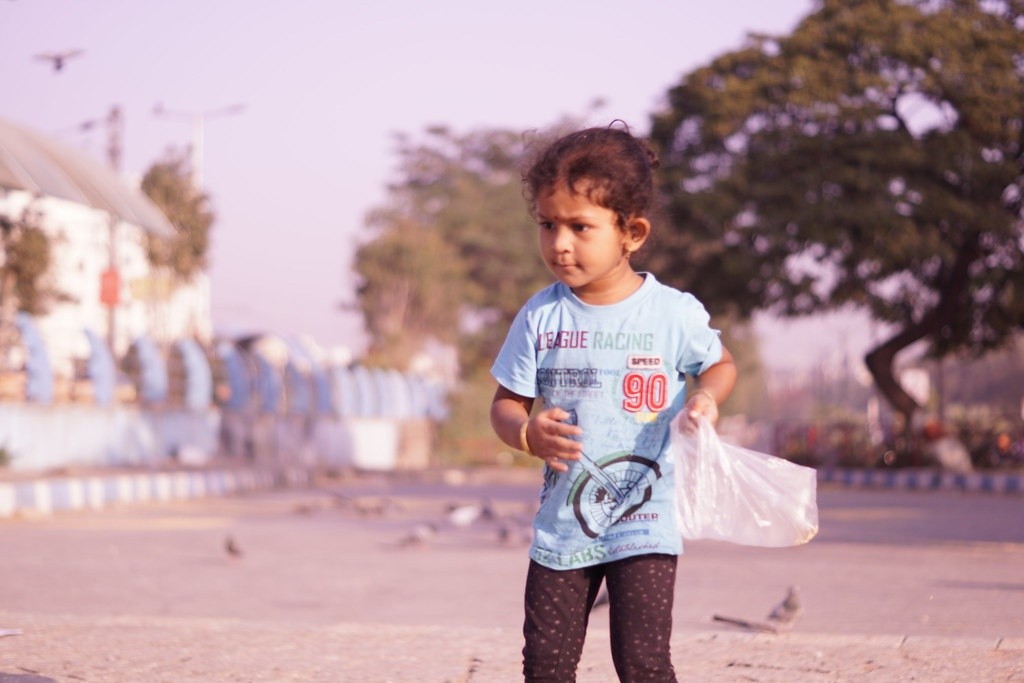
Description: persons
491,119,737,683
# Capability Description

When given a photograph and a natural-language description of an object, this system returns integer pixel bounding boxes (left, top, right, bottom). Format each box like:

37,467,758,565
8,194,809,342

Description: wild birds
222,482,535,559
712,584,803,635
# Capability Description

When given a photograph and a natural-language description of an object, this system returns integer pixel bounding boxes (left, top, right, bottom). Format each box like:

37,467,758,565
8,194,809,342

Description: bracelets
685,390,712,404
520,420,536,457
